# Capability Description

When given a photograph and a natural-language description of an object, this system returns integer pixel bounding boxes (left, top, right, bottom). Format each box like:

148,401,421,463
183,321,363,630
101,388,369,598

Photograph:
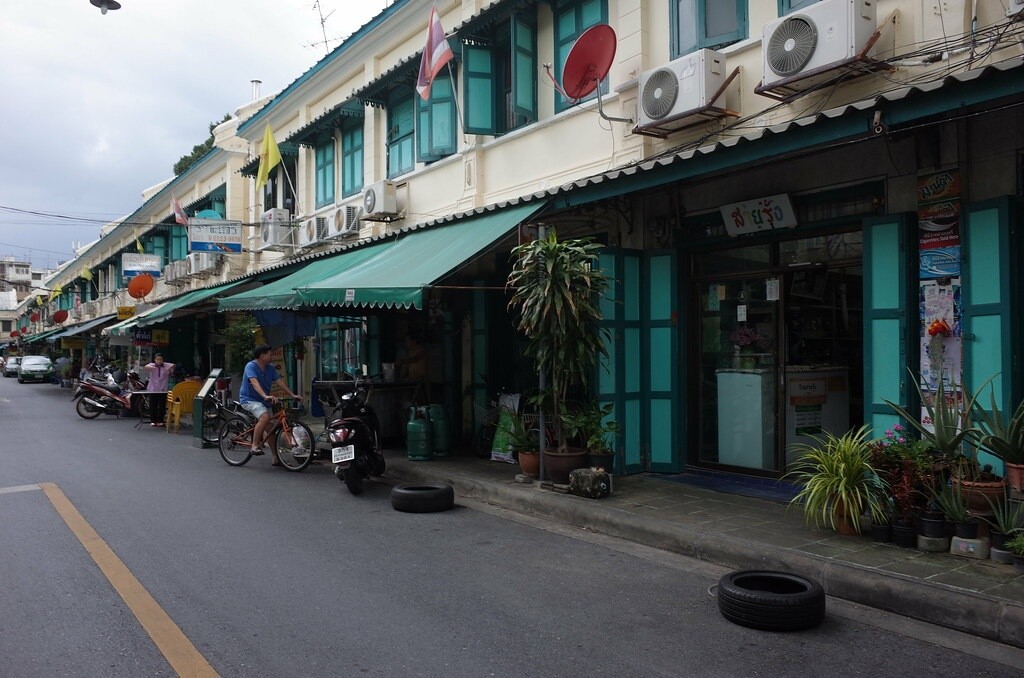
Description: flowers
925,317,950,385
729,328,758,348
874,423,908,455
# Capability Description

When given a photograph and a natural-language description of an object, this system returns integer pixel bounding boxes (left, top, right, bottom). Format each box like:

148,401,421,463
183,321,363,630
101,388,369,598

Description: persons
395,332,428,379
91,347,108,368
239,344,303,466
144,353,175,426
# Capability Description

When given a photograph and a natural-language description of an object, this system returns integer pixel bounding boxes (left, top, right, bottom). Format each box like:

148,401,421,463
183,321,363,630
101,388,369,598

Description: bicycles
202,390,242,443
217,395,316,473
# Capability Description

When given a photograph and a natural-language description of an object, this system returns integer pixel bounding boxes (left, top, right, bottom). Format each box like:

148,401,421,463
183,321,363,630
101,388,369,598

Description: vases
737,343,754,370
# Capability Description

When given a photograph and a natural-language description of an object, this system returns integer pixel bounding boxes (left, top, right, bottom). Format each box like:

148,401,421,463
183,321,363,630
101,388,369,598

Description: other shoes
157,423,164,426
150,423,156,425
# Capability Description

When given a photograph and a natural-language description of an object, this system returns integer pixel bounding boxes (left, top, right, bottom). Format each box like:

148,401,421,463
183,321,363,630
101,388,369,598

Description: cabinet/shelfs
786,273,864,361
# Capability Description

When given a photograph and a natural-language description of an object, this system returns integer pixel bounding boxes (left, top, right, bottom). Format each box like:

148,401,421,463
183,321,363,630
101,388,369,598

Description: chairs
164,380,204,434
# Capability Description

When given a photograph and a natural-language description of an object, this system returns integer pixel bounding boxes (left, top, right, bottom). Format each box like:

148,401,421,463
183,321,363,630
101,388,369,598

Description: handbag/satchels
292,427,311,455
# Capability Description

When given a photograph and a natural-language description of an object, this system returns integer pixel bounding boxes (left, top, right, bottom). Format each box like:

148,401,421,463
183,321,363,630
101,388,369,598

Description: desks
128,389,168,431
714,365,850,470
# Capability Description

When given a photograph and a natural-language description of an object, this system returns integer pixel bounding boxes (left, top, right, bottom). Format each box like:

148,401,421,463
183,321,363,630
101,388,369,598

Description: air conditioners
70,307,80,319
640,51,726,133
362,180,397,221
256,207,289,249
761,0,877,96
329,206,360,240
163,246,225,286
299,216,326,247
82,301,94,316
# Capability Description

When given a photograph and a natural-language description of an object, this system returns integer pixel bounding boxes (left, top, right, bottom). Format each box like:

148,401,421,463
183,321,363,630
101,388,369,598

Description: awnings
22,315,117,343
101,277,251,336
291,198,554,310
216,238,400,312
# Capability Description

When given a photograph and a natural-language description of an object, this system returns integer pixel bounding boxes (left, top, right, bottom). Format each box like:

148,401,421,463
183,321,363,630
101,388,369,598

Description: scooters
318,363,387,496
70,371,154,420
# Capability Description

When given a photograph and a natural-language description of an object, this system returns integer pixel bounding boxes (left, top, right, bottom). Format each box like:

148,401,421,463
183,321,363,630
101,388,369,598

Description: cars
17,354,56,384
2,356,23,377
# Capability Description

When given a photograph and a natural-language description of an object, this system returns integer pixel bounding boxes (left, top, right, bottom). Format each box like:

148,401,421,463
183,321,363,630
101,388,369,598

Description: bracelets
400,360,403,364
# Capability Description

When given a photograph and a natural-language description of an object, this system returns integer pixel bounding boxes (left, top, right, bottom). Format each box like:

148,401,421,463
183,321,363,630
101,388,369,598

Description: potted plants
60,364,73,387
565,398,624,473
787,408,1024,563
497,404,545,478
506,225,610,480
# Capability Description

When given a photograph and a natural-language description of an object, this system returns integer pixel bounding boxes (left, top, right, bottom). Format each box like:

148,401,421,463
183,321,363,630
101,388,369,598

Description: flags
134,230,143,253
416,2,455,101
81,263,94,280
172,193,188,226
256,123,281,191
48,283,62,302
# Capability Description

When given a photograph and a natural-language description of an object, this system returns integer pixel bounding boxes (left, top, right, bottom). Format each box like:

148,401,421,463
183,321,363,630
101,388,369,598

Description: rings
276,398,277,399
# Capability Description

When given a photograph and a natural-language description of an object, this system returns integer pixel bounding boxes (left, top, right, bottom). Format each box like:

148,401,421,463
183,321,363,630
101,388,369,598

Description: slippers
250,447,265,455
272,460,290,466
